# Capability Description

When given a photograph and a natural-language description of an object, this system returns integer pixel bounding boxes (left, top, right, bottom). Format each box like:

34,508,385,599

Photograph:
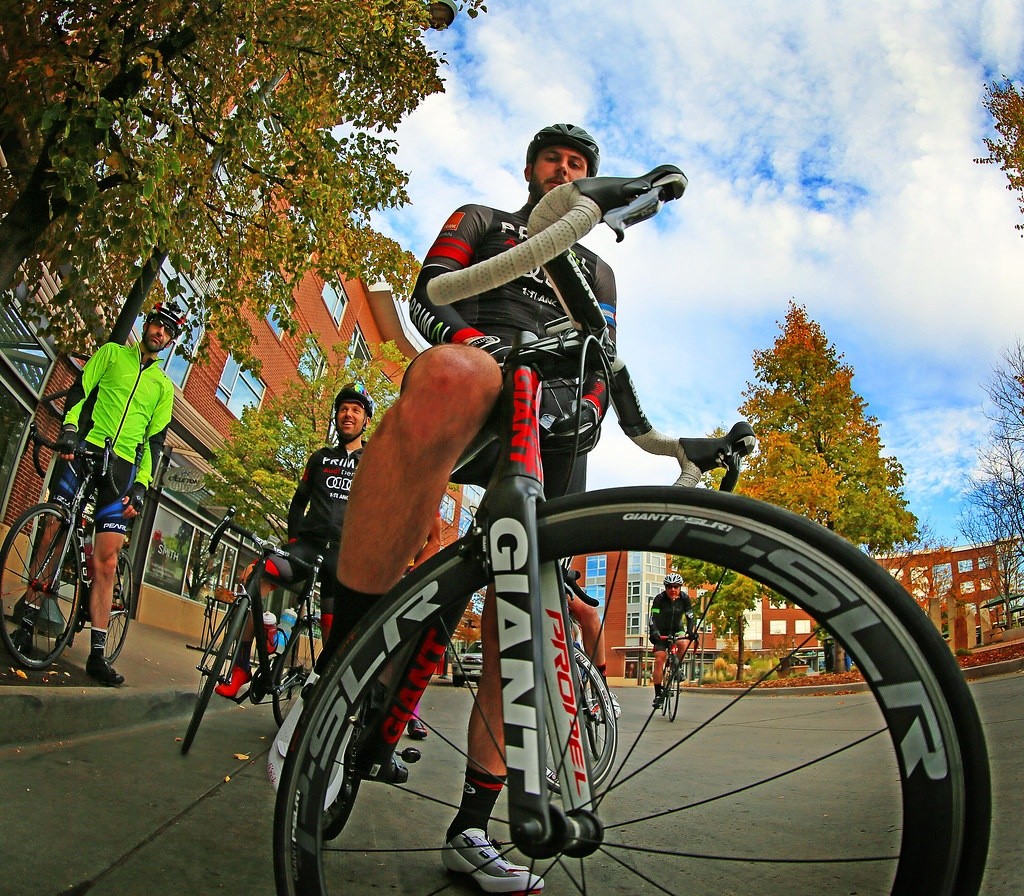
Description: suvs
450,639,484,687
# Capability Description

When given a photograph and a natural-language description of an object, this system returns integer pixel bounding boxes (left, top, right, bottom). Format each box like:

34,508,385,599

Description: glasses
148,318,175,339
666,585,681,590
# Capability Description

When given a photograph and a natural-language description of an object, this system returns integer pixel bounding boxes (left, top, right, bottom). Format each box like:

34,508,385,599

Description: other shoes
674,669,686,680
215,664,253,698
599,690,621,721
652,695,662,708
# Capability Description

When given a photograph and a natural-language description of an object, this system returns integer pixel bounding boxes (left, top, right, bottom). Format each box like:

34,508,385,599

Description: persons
649,571,698,705
3,301,187,688
214,383,374,699
546,550,622,724
269,123,616,896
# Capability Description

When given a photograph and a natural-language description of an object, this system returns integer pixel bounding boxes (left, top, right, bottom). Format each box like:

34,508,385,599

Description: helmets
664,574,683,586
335,383,374,419
147,302,185,339
526,124,600,177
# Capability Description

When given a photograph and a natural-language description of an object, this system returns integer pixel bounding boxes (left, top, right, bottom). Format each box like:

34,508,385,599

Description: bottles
262,611,278,654
273,606,298,654
85,535,96,576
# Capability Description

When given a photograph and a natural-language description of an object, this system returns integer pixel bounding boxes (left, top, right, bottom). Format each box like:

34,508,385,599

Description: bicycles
570,639,615,764
180,502,334,754
266,163,995,895
649,631,700,722
0,421,138,672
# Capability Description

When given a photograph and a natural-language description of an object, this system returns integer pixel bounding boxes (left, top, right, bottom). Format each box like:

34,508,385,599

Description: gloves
465,335,511,371
649,635,660,644
538,399,601,458
685,630,693,641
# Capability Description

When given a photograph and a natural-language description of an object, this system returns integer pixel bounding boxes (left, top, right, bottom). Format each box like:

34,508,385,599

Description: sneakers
267,666,321,794
14,630,32,653
441,829,544,892
408,718,429,738
86,655,124,682
